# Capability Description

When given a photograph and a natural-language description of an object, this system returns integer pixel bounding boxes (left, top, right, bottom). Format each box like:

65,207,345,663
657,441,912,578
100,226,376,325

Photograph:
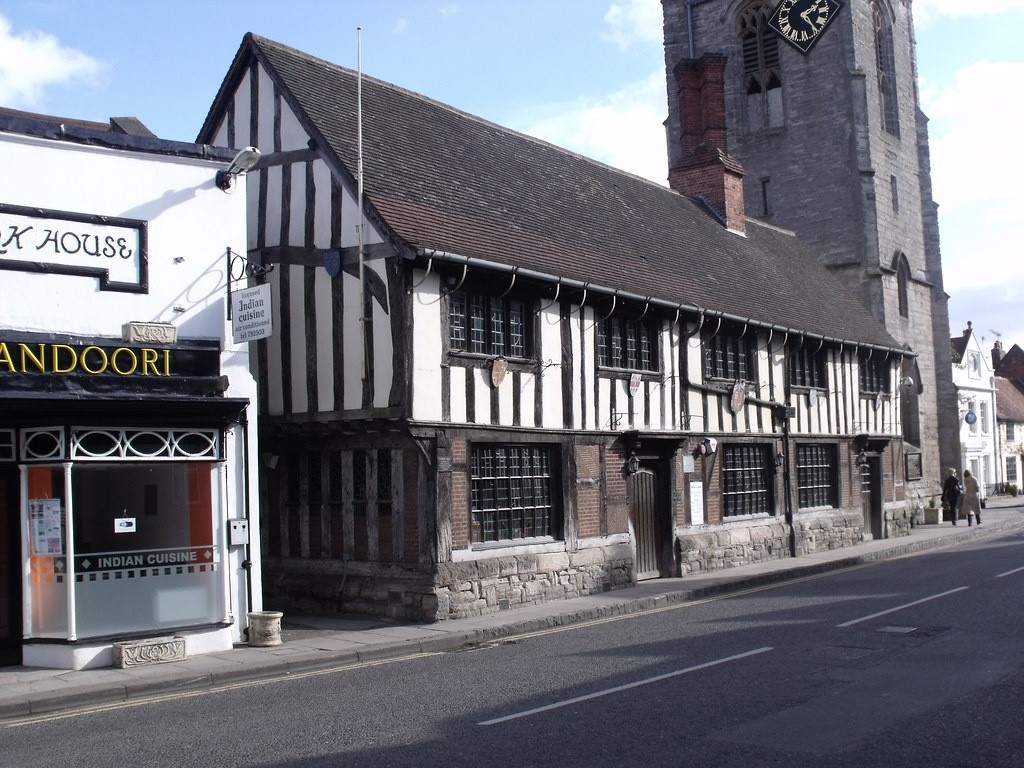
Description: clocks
764,0,844,57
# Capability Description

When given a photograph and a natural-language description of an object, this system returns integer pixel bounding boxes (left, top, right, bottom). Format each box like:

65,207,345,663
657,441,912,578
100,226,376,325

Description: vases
247,610,283,646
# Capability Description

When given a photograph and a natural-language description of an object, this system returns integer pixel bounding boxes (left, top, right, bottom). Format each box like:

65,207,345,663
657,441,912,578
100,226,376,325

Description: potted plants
924,494,943,524
1010,484,1018,497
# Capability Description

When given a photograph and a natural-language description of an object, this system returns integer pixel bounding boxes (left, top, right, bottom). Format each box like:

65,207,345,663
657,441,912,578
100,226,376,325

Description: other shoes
953,522,956,525
977,521,980,524
969,522,971,526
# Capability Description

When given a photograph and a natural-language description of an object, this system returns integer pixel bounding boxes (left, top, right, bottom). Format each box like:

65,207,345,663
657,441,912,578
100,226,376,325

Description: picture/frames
905,452,923,481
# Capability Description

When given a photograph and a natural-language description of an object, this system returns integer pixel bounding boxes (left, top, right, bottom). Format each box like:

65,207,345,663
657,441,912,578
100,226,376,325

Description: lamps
856,449,868,466
625,451,642,478
774,451,785,475
958,407,977,425
216,146,262,196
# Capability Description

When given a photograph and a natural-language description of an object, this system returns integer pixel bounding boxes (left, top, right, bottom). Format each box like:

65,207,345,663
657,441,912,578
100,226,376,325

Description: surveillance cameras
905,377,914,387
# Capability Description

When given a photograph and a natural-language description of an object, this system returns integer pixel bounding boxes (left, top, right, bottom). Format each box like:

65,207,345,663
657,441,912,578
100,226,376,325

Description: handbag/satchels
941,495,949,509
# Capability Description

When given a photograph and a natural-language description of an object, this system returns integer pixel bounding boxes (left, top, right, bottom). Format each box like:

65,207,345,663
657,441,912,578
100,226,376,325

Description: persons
963,470,983,527
940,468,964,526
